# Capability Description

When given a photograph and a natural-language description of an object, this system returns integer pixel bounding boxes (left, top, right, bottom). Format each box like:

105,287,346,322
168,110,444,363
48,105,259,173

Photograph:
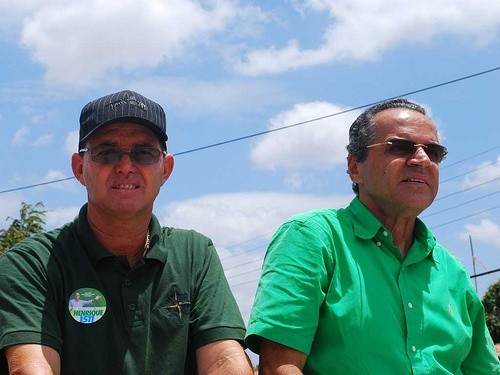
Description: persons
0,89,256,375
243,98,500,375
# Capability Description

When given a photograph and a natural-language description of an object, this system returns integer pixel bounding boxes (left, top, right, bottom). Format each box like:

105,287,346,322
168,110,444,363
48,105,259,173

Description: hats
78,90,169,144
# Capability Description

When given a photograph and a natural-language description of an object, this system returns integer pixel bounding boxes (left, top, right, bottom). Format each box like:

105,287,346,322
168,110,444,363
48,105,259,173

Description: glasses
360,139,448,164
78,145,168,166
